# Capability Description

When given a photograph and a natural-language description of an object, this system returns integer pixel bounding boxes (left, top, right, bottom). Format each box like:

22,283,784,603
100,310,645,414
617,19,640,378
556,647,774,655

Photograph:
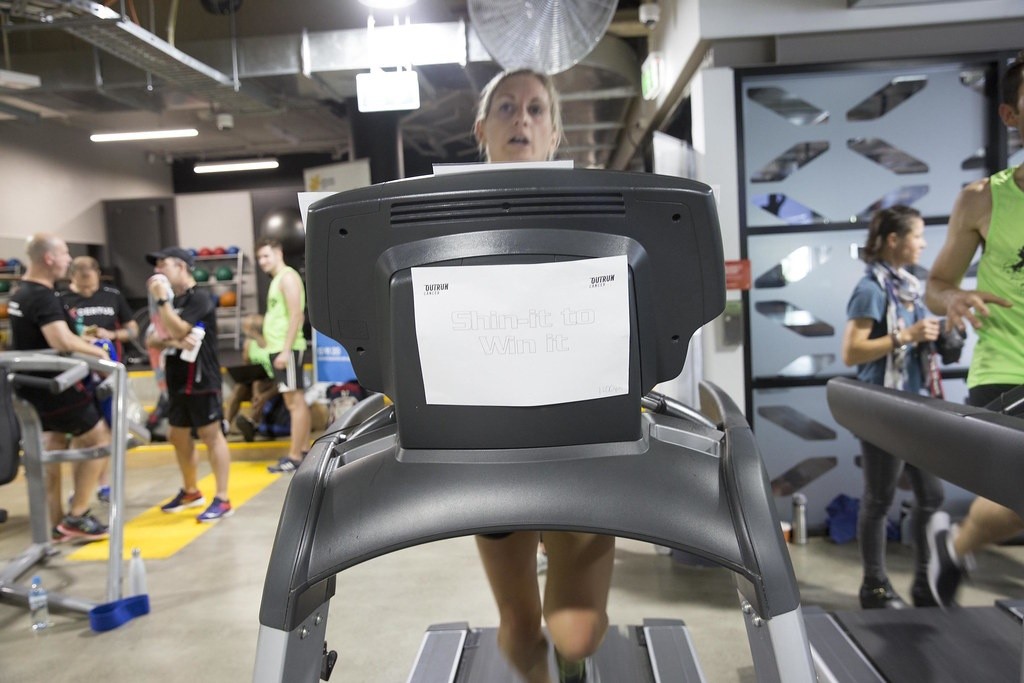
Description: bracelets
892,330,906,350
112,330,117,341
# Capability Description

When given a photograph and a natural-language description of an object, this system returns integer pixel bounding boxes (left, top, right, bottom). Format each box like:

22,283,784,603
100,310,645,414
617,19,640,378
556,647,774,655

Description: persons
59,257,138,503
837,202,964,609
923,56,1024,610
254,238,311,473
9,234,110,543
144,247,232,520
473,67,615,683
222,314,276,440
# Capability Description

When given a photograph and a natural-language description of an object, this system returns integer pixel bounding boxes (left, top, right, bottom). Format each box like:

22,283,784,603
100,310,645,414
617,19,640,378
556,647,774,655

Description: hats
144,246,195,267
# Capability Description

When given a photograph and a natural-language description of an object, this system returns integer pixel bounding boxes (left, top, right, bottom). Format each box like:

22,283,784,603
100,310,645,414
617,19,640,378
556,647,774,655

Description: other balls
186,246,239,308
0,259,27,346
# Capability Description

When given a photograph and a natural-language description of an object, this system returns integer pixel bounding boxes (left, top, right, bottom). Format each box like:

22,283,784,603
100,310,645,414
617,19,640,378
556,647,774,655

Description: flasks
792,493,808,545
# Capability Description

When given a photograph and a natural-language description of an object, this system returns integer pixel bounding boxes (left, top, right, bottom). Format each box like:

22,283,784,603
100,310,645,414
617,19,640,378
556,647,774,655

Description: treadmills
254,167,1024,683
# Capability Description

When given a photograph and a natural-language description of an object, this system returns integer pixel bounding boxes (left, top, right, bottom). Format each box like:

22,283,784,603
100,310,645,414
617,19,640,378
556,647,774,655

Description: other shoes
235,415,260,440
148,428,167,441
860,577,911,609
553,644,594,683
97,487,109,504
912,505,977,610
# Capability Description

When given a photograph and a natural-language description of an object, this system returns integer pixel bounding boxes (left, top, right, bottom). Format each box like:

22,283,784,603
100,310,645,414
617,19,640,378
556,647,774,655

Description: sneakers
163,489,204,512
198,496,234,522
267,455,303,471
51,509,111,543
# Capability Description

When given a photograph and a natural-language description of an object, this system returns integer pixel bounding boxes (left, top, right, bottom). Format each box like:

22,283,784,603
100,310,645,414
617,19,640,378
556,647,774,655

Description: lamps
88,126,199,143
192,129,279,174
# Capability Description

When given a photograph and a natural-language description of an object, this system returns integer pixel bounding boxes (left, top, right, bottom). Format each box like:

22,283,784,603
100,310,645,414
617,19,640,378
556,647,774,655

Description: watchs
156,299,169,306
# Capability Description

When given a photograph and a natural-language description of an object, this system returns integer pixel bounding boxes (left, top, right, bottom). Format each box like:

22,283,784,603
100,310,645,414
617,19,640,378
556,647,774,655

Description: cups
781,523,792,542
937,320,963,348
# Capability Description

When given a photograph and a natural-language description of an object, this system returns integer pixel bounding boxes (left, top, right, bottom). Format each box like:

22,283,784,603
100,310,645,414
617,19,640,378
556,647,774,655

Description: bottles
920,340,943,400
180,322,207,362
129,549,147,596
29,577,47,630
